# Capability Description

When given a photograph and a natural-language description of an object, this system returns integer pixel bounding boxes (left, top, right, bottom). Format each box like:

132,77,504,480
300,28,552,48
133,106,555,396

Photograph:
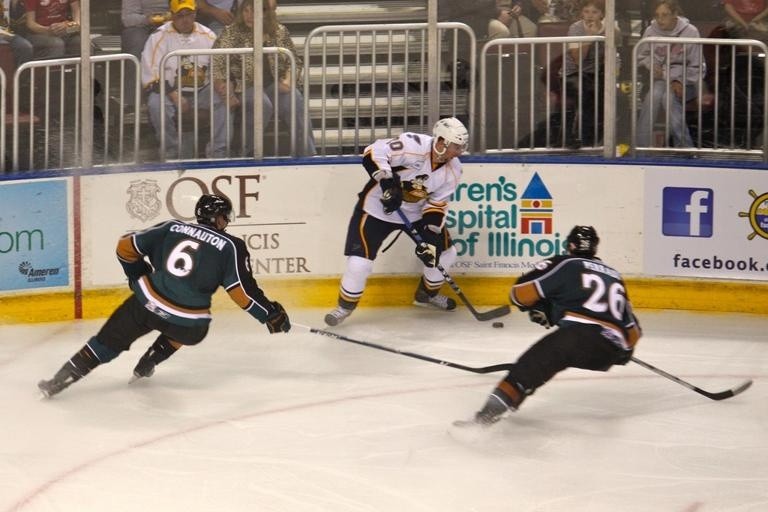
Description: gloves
379,177,403,214
415,229,442,268
528,309,551,329
266,301,291,333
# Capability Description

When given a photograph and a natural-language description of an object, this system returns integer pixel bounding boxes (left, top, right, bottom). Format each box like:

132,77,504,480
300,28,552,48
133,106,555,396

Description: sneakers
415,287,456,310
324,306,352,326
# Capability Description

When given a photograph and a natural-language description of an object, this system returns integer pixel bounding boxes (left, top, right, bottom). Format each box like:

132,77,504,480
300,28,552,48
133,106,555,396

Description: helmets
568,225,600,259
195,194,235,226
432,117,470,164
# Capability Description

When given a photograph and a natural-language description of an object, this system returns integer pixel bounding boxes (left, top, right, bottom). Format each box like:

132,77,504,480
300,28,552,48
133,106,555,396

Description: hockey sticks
394,208,510,321
290,321,516,375
630,355,752,401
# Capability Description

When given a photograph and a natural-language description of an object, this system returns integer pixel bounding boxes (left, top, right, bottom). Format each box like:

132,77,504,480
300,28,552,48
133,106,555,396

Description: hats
168,0,196,14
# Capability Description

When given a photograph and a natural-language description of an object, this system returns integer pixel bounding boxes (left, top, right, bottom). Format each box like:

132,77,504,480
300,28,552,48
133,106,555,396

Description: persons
4,1,314,155
325,117,470,326
38,194,290,402
448,227,642,440
443,0,768,153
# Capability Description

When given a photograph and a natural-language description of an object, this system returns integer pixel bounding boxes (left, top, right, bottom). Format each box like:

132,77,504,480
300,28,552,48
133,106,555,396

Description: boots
38,360,82,398
133,346,162,378
451,405,507,427
